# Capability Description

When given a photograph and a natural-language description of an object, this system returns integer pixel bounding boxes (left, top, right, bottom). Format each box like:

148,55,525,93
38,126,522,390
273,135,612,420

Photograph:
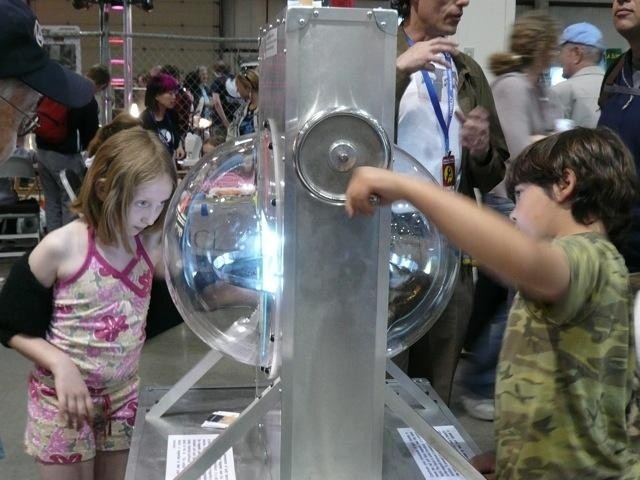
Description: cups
554,117,577,133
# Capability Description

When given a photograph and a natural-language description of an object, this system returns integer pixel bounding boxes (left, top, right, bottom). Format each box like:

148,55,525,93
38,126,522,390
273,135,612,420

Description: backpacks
174,132,204,170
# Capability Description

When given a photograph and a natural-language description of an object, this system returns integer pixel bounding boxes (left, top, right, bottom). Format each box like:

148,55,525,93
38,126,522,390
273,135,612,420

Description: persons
32,63,108,228
1,125,183,480
457,9,564,423
141,60,260,193
345,125,631,479
598,0,640,465
544,21,606,129
0,0,98,167
394,0,510,409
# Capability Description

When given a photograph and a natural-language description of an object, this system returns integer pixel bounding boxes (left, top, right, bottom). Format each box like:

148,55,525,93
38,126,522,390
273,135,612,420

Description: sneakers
458,389,496,422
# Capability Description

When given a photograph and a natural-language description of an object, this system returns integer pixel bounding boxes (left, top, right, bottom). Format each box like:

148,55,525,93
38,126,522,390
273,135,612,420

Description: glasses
1,98,42,137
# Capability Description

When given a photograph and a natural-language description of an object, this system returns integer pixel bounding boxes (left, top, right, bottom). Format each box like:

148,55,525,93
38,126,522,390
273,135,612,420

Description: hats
556,20,607,51
0,0,97,109
